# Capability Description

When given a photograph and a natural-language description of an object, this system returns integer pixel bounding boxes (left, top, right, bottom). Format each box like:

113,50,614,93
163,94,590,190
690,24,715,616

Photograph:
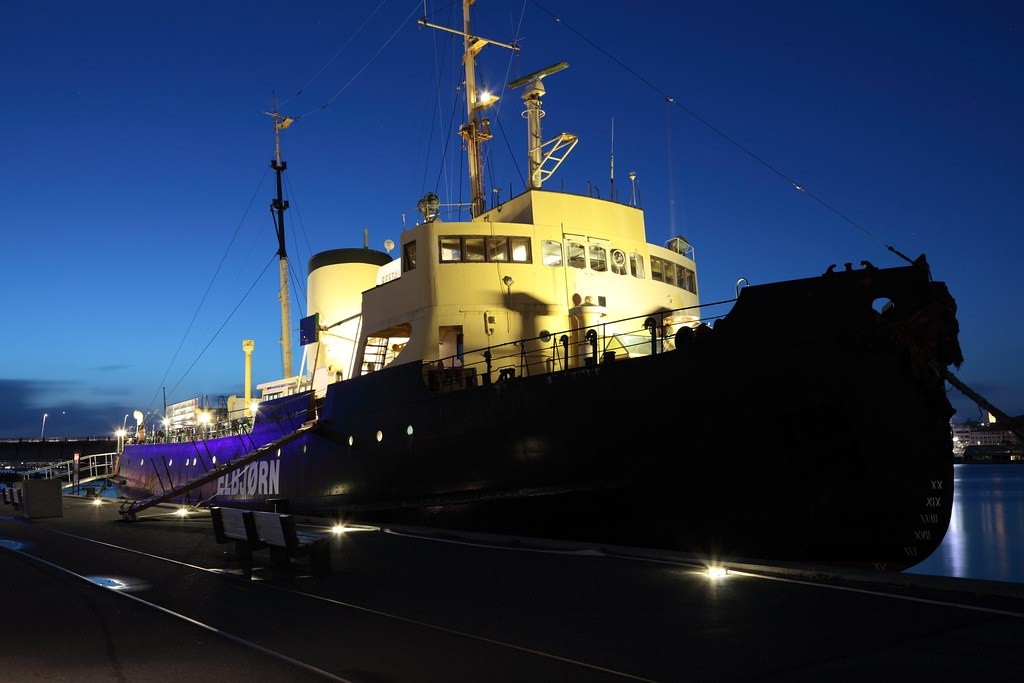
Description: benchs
211,505,331,580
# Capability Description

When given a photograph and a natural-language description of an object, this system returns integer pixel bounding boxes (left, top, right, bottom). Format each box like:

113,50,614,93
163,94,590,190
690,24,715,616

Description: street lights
40,413,48,439
264,111,300,373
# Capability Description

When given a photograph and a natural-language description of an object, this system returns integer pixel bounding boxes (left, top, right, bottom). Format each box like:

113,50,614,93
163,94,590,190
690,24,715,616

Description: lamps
502,277,514,297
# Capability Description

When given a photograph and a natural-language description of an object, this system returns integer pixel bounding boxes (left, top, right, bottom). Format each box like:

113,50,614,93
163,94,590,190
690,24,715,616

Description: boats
106,0,965,582
